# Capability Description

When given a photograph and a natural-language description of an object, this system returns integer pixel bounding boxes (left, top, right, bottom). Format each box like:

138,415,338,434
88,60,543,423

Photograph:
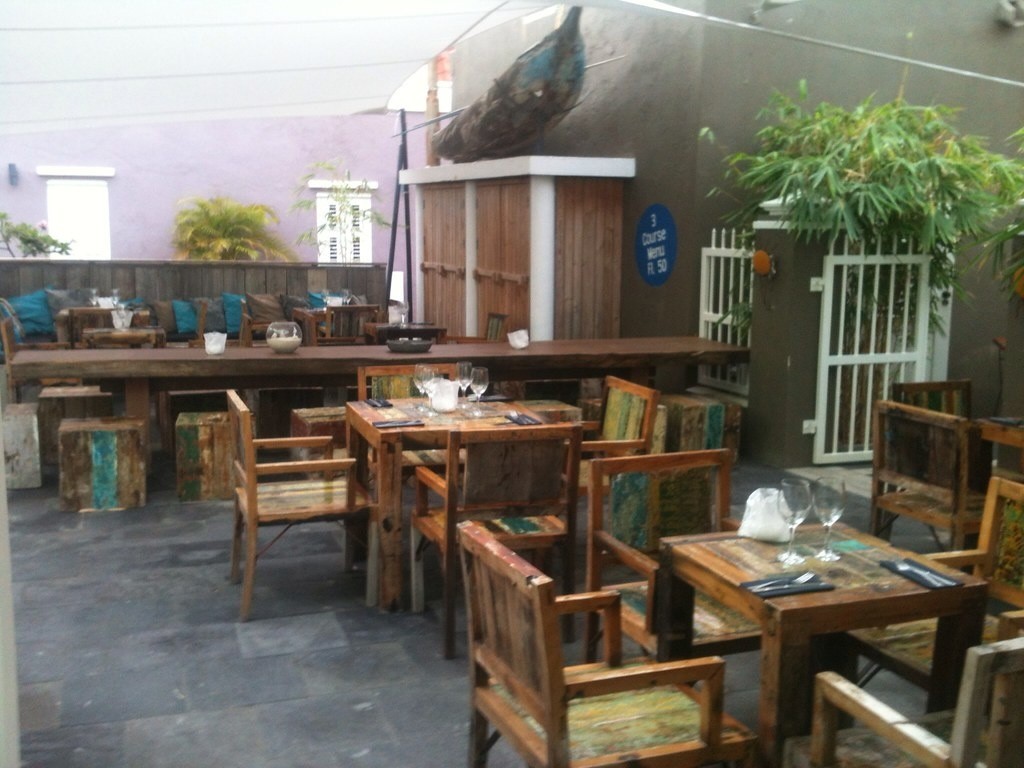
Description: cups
97,296,118,307
111,288,120,310
87,289,99,307
110,310,133,328
320,286,352,306
115,303,131,330
203,332,227,355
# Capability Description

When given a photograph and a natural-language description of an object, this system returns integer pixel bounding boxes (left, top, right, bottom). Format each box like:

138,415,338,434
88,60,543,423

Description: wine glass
812,475,846,562
413,363,438,418
454,361,490,418
776,478,812,566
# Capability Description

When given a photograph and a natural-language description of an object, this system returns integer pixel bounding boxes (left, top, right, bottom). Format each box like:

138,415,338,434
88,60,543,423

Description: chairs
227,390,1024,768
0,299,512,454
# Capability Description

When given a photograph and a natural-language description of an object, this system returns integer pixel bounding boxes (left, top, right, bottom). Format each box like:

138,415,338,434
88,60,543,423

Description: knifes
911,564,957,587
751,582,831,593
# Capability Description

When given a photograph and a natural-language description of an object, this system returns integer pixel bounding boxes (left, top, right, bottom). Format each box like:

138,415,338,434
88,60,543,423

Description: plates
385,339,434,353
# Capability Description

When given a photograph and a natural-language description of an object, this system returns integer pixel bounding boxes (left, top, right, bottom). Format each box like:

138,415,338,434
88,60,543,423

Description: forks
892,552,942,587
747,570,816,590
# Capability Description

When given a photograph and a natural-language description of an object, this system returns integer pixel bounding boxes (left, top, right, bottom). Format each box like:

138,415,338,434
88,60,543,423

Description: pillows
1,285,370,339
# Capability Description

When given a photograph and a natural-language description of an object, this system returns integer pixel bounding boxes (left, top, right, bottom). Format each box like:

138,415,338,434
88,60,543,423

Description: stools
2,401,47,490
176,410,258,500
37,386,113,466
57,418,147,510
291,407,352,480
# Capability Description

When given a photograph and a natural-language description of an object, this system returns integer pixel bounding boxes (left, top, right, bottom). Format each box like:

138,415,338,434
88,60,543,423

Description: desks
346,398,583,613
83,329,168,440
659,524,990,767
976,415,1024,479
368,323,447,344
